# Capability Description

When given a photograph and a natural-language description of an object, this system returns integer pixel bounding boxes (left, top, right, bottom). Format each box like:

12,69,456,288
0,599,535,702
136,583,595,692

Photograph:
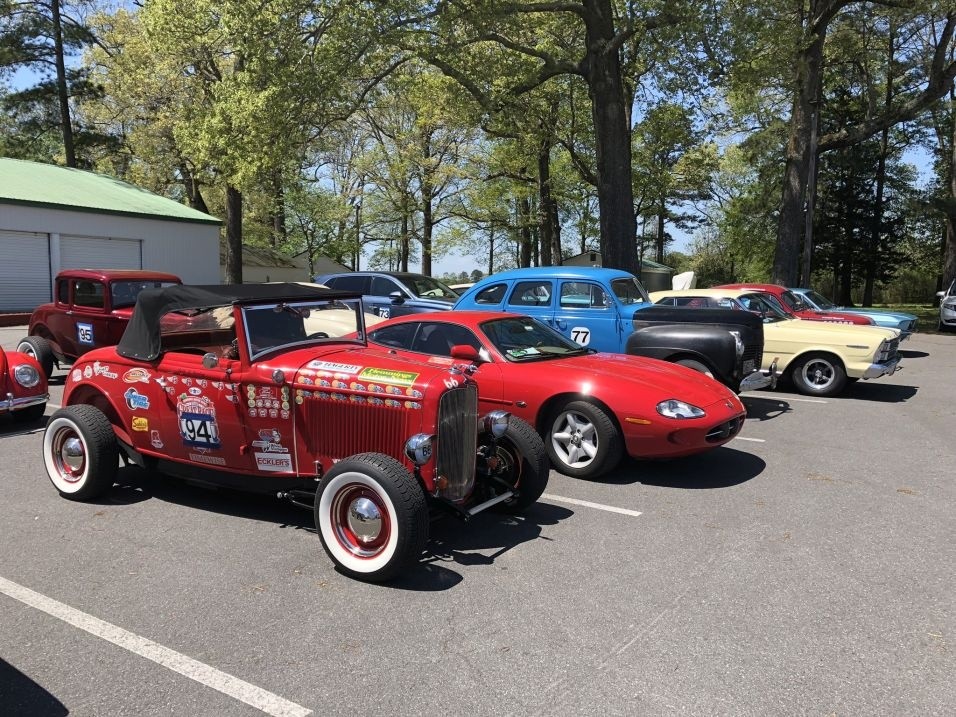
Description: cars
935,277,956,332
41,280,551,583
210,264,921,400
15,267,236,383
0,341,50,433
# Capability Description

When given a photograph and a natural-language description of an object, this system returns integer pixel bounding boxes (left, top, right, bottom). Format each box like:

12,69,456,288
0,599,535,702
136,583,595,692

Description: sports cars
335,308,748,480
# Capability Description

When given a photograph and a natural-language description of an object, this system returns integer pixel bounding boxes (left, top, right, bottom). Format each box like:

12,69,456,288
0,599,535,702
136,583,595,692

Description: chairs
77,282,96,306
245,310,306,347
113,283,130,304
